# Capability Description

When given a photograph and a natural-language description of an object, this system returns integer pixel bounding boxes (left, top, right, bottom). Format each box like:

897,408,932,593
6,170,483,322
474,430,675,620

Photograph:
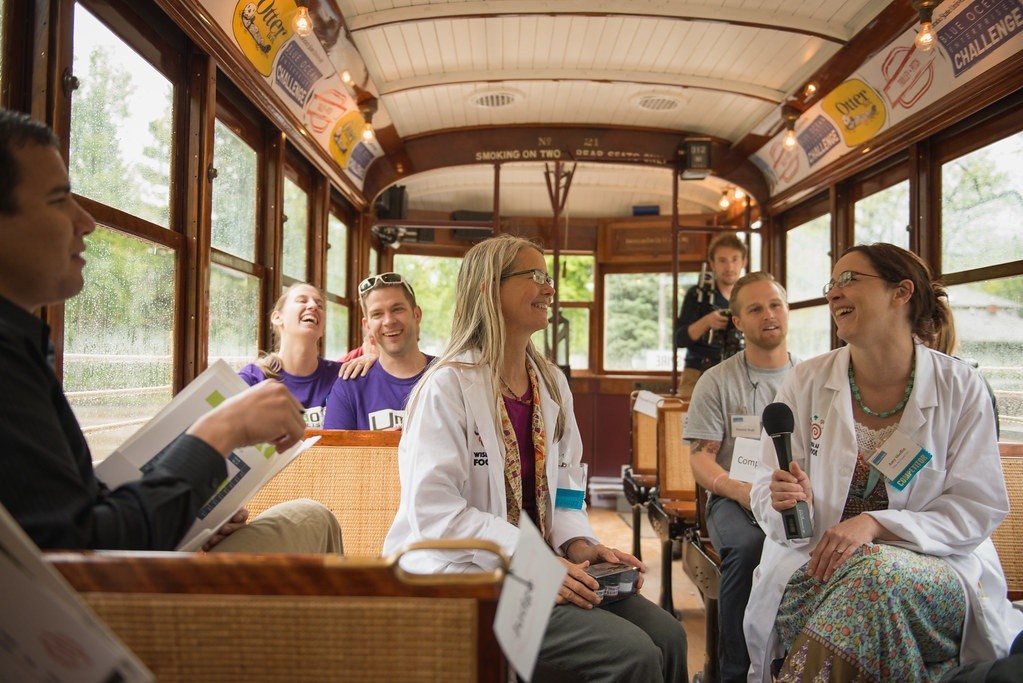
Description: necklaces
493,369,533,405
848,357,916,418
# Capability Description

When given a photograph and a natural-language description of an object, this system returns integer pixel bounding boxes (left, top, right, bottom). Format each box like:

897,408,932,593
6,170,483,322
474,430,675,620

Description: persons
0,104,344,555
681,271,807,683
324,271,439,431
238,282,379,430
745,242,1023,683
676,233,748,396
381,237,690,683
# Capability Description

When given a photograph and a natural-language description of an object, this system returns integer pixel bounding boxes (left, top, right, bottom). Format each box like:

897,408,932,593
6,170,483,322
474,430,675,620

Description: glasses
493,268,554,289
358,272,413,304
823,270,898,296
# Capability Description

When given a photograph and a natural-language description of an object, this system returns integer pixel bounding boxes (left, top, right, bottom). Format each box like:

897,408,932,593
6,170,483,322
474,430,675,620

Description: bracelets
712,473,728,494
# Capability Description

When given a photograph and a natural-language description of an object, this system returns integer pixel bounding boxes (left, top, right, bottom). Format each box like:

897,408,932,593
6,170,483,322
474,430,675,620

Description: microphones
763,403,813,540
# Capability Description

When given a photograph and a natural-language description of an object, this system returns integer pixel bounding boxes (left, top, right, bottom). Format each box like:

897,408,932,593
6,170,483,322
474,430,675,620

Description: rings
834,549,843,554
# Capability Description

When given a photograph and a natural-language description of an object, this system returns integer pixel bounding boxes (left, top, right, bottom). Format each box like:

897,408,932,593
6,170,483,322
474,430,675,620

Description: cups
591,569,639,602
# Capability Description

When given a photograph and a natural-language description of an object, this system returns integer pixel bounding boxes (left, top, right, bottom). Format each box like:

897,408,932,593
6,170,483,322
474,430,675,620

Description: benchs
41,428,520,683
621,390,1023,683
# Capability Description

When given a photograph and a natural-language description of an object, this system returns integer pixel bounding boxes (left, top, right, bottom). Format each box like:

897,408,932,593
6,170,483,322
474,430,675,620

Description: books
95,360,321,551
